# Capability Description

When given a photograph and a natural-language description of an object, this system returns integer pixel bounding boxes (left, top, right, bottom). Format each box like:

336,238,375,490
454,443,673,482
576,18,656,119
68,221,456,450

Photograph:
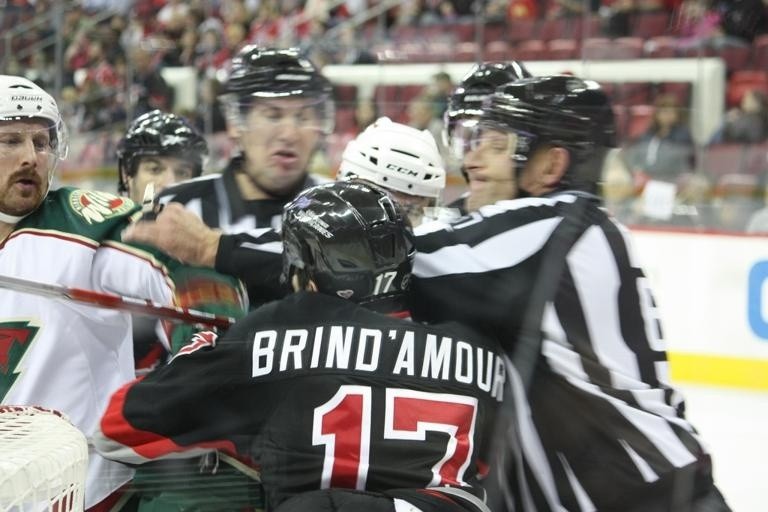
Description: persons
0,1,768,512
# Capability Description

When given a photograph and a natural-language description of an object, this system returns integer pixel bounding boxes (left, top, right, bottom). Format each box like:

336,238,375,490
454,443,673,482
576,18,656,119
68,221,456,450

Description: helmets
217,43,336,135
0,75,69,158
117,109,207,191
335,116,447,226
277,178,416,311
443,58,617,196
